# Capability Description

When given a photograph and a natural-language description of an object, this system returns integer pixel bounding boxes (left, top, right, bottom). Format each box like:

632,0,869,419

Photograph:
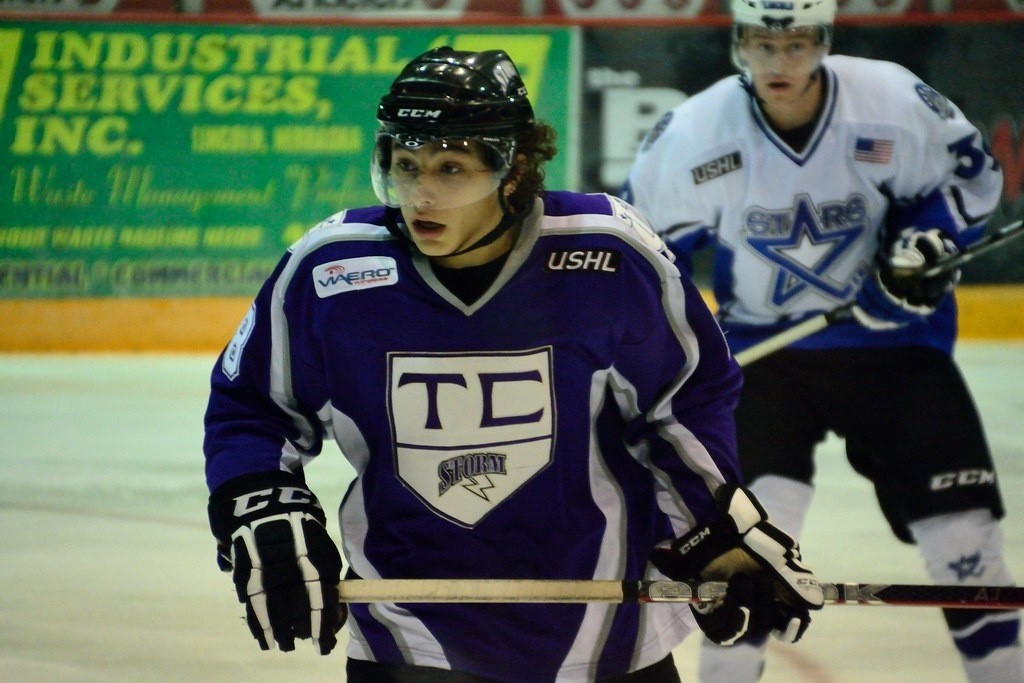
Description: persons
613,0,1024,682
202,44,825,683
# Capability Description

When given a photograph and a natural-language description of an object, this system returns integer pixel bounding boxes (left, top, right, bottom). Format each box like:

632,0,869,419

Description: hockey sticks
335,579,1023,610
734,222,1023,369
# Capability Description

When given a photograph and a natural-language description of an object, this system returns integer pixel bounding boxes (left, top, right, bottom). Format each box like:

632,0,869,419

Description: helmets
725,0,837,49
376,47,535,174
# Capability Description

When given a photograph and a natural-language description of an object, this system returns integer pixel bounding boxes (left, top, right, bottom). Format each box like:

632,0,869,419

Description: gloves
875,195,962,313
652,481,825,647
207,469,348,656
